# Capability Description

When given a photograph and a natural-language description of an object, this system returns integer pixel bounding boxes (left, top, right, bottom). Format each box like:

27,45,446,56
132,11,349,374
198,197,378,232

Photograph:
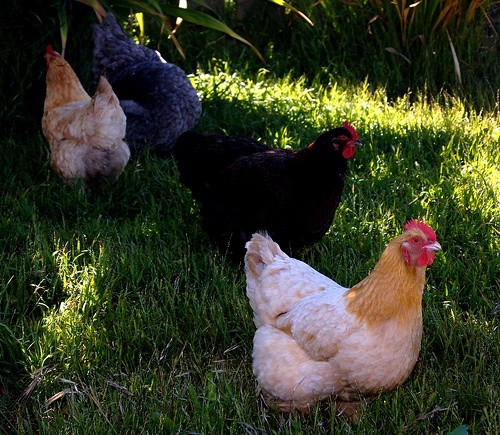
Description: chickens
244,216,441,425
41,43,129,183
91,12,203,148
174,120,363,261
